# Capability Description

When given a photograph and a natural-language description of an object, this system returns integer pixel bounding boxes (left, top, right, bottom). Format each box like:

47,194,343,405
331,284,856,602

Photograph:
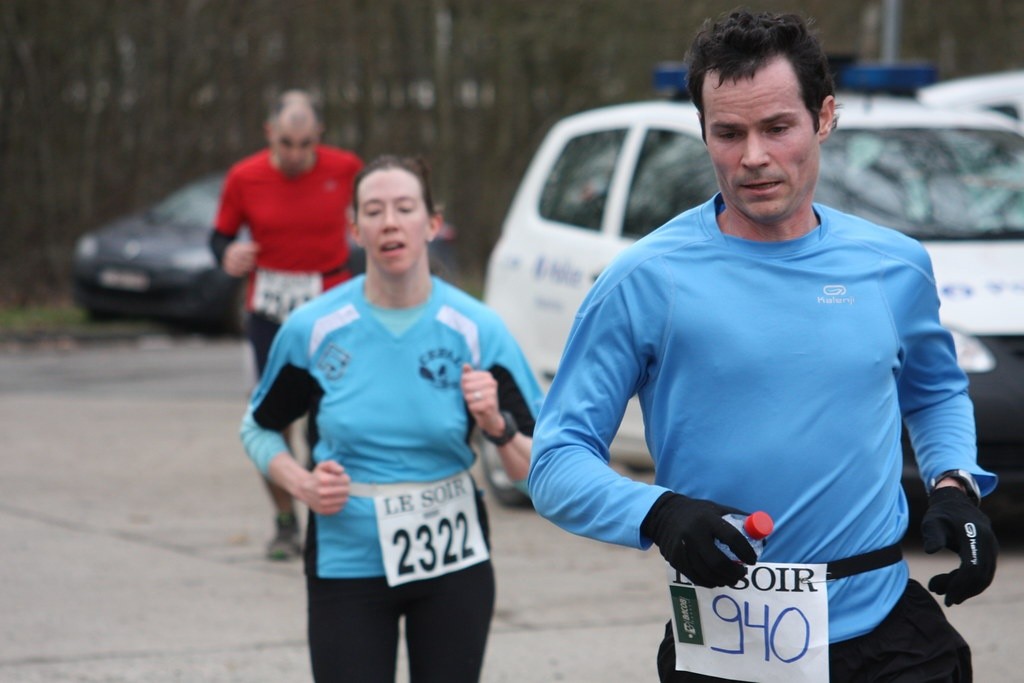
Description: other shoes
269,512,301,560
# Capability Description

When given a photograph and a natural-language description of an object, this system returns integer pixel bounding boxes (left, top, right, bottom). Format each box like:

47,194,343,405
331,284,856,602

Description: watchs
931,469,981,507
481,413,519,446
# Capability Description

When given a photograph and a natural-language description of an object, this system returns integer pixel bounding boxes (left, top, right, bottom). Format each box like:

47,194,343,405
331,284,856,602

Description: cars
914,72,1024,132
64,172,463,321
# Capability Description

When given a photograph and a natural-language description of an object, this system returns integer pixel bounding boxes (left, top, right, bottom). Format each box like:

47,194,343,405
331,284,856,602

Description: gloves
641,491,768,588
921,486,999,607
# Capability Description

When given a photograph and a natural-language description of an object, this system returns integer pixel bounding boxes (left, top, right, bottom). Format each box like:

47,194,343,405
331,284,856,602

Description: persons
206,85,365,561
524,9,1002,683
239,157,542,673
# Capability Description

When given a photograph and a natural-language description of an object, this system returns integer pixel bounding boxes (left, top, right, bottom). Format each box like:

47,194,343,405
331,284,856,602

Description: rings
475,391,482,401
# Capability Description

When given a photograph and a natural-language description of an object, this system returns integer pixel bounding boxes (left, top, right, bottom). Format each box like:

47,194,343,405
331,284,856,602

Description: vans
473,57,1023,538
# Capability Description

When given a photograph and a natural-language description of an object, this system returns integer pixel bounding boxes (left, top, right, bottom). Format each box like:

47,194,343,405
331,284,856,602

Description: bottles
715,511,773,567
